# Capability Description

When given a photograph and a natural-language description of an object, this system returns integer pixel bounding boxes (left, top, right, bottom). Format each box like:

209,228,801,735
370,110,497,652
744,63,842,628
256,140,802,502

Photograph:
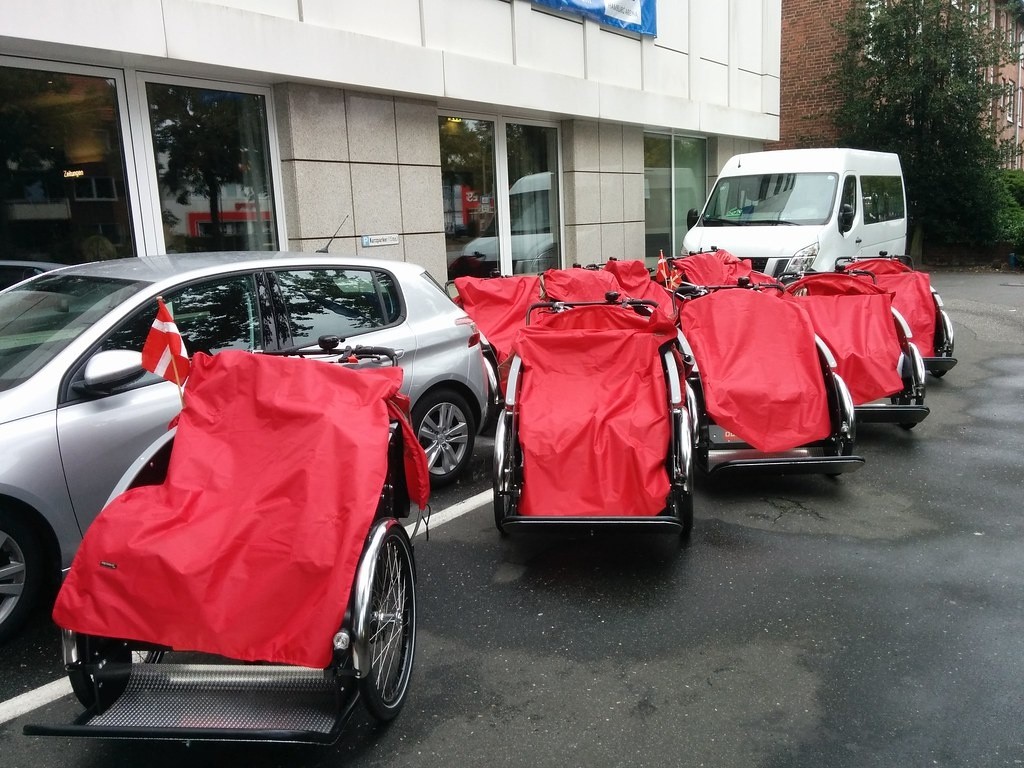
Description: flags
656,254,671,283
668,268,683,291
141,300,191,386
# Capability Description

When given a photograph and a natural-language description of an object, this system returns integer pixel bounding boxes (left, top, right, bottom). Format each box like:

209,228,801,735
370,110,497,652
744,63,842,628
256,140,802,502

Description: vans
460,164,701,278
681,146,908,285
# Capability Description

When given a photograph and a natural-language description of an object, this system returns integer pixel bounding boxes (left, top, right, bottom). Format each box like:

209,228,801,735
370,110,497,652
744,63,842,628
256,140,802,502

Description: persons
163,222,178,254
81,236,117,262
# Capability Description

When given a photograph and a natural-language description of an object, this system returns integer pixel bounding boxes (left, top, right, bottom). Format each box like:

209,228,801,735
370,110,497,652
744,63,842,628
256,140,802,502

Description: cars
0,253,492,663
0,260,84,337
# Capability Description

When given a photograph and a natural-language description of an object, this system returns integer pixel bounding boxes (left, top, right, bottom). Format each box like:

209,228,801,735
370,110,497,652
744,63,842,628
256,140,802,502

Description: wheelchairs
444,257,958,547
20,336,418,749
